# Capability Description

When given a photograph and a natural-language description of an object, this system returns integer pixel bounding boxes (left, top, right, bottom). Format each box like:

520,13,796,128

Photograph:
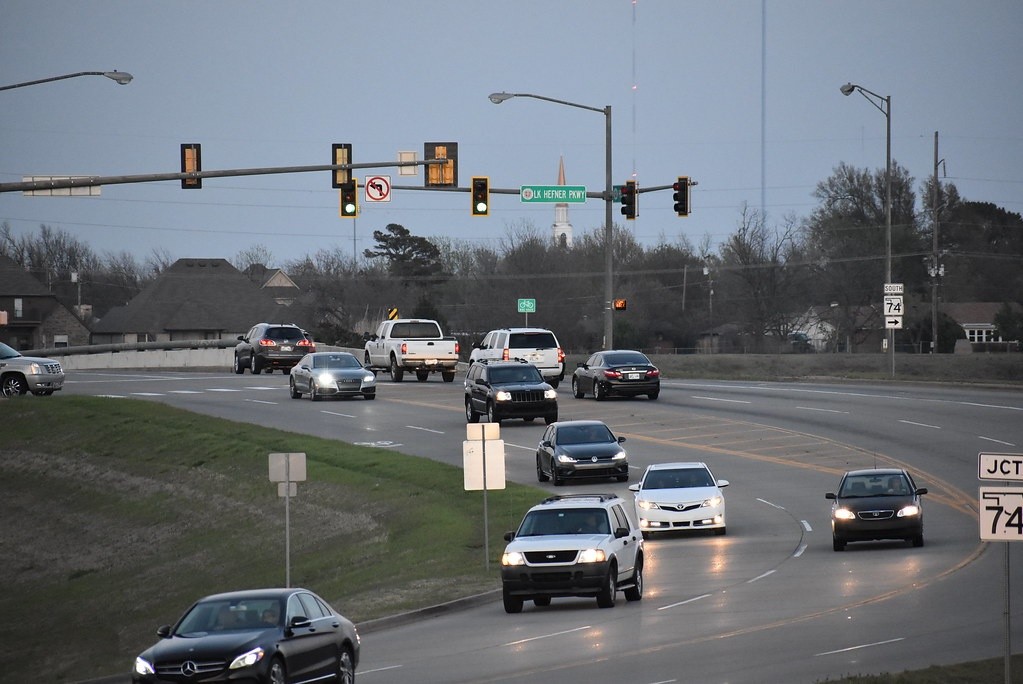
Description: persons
582,512,607,533
888,477,907,495
262,610,277,622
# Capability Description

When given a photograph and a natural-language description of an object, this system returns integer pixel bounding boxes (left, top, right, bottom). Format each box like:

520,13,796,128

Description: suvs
501,493,644,614
234,322,318,375
467,327,566,389
464,357,558,428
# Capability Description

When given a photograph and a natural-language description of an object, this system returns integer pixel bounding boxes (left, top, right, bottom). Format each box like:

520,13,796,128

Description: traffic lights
471,176,489,217
340,178,358,218
673,177,688,217
621,182,635,220
613,299,626,310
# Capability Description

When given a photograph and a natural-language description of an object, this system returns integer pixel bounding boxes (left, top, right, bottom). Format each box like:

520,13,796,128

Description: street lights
840,82,896,376
488,90,612,351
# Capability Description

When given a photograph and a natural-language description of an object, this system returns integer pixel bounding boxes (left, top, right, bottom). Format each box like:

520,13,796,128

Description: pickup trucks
364,318,460,382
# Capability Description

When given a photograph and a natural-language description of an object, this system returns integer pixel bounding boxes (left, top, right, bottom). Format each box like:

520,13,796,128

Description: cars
289,352,376,401
825,468,928,551
132,588,361,684
0,342,65,398
629,461,729,540
572,350,661,401
536,420,629,486
